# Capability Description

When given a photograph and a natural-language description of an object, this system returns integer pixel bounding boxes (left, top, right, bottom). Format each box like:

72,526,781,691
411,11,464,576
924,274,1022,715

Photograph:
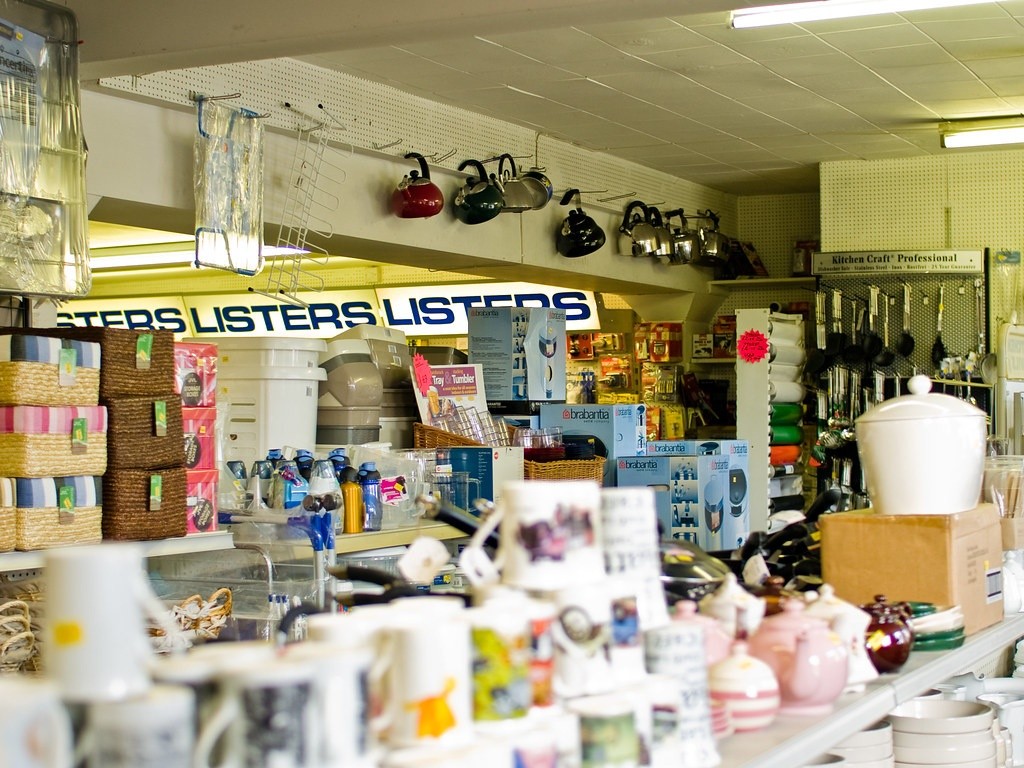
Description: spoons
966,372,978,407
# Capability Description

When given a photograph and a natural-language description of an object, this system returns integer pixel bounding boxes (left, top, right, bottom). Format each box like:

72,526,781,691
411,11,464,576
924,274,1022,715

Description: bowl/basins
801,689,1017,768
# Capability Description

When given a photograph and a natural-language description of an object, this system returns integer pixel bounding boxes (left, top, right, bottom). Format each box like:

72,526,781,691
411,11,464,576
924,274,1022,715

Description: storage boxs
170,324,468,535
819,504,1004,636
467,307,749,551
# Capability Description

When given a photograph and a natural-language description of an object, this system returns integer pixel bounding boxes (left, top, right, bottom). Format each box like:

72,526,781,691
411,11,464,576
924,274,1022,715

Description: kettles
665,208,700,265
649,207,675,257
391,153,444,218
519,167,553,210
452,160,502,224
556,189,606,258
490,154,534,211
702,210,730,268
618,201,661,257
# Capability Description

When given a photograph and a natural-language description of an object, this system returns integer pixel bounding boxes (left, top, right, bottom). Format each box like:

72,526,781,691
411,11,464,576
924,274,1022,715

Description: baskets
0,578,44,676
147,588,233,643
0,326,188,553
413,421,607,488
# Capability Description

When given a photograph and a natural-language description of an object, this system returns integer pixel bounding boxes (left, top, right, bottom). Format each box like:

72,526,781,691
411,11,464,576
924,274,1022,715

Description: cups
434,407,562,448
349,445,480,528
855,376,987,514
0,479,783,768
1004,551,1024,619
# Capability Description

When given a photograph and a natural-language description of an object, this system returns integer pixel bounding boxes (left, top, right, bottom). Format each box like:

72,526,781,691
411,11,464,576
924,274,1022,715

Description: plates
912,602,966,650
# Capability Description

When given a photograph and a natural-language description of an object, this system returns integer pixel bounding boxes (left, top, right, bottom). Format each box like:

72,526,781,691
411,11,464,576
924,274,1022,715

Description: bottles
328,448,350,483
266,449,286,469
218,460,247,524
294,449,313,481
309,461,345,534
250,461,274,507
358,462,382,530
276,461,309,509
341,467,364,533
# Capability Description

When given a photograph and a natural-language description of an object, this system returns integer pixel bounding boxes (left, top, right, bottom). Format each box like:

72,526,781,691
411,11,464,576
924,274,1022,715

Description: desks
719,612,1024,768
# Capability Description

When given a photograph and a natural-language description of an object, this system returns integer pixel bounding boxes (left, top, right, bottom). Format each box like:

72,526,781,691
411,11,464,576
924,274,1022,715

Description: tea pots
750,598,846,712
701,571,764,636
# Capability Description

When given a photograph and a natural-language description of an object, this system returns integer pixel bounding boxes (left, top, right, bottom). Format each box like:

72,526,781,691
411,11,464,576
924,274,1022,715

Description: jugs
937,684,966,701
976,693,1024,768
